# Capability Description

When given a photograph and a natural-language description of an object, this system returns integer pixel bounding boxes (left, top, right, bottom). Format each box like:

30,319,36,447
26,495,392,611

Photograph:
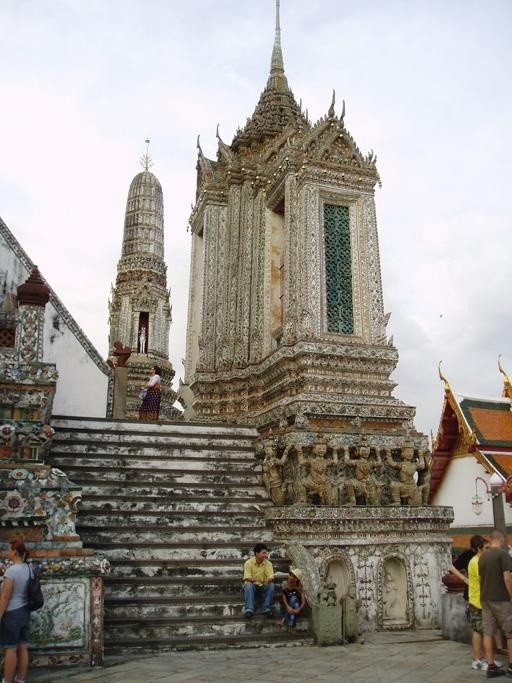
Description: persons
261,431,432,508
139,366,161,423
339,583,365,644
278,568,306,628
449,530,512,678
383,573,398,618
242,542,276,621
0,538,34,683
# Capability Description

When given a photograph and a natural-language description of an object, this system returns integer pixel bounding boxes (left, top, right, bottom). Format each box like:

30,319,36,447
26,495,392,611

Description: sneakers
471,655,512,677
245,611,274,618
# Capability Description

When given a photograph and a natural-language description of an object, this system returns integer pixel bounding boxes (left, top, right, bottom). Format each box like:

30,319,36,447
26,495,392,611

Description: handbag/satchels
25,560,44,611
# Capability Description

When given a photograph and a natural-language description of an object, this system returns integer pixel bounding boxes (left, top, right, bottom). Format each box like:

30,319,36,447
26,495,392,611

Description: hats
290,568,302,579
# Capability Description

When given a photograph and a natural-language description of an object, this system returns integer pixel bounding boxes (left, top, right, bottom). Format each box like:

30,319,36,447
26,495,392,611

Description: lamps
471,477,492,516
